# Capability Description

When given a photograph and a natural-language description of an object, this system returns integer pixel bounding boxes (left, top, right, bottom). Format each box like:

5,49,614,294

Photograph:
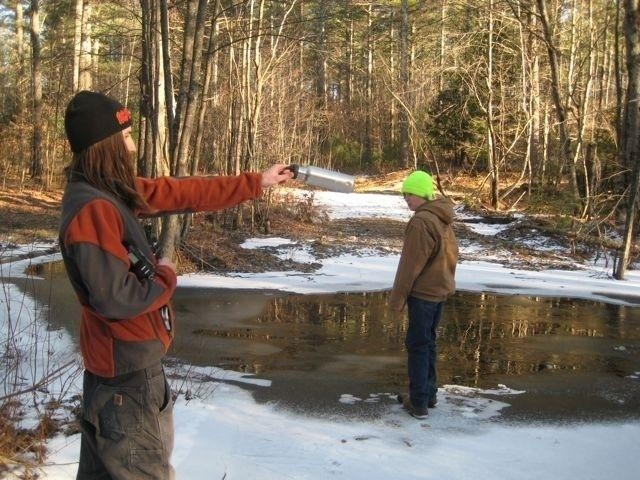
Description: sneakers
397,388,440,420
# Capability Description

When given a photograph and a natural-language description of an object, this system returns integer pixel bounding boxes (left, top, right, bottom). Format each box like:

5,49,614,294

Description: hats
401,170,436,203
64,89,135,153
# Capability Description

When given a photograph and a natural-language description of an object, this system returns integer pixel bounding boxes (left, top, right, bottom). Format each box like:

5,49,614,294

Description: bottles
282,164,355,192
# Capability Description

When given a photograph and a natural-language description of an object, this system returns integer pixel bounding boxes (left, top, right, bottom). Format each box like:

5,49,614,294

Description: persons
389,171,460,419
58,91,299,480
